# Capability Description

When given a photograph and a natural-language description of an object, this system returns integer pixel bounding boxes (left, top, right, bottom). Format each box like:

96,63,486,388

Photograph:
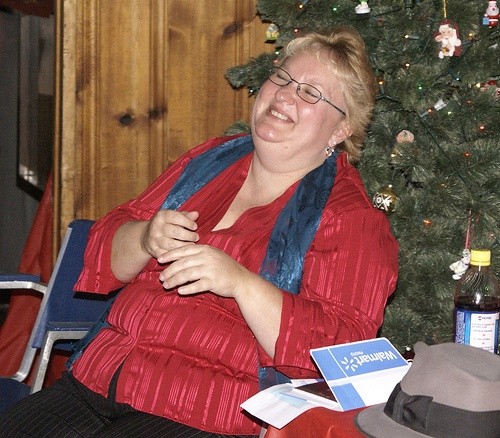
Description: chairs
0,219,124,395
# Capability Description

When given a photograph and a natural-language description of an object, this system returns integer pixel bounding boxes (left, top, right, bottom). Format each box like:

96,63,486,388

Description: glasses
268,66,346,115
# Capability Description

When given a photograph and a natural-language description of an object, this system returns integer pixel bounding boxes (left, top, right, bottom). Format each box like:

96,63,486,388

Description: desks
261,380,371,438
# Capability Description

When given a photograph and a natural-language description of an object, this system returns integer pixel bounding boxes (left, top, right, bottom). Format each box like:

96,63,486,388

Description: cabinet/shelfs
52,0,280,268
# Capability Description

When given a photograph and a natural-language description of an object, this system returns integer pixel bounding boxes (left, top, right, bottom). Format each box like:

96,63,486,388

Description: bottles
454,249,500,354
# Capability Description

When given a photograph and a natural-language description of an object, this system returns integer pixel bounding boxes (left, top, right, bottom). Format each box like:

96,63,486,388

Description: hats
354,342,500,438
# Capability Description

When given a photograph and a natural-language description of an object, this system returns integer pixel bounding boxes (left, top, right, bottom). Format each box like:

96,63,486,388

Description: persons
0,25,400,438
